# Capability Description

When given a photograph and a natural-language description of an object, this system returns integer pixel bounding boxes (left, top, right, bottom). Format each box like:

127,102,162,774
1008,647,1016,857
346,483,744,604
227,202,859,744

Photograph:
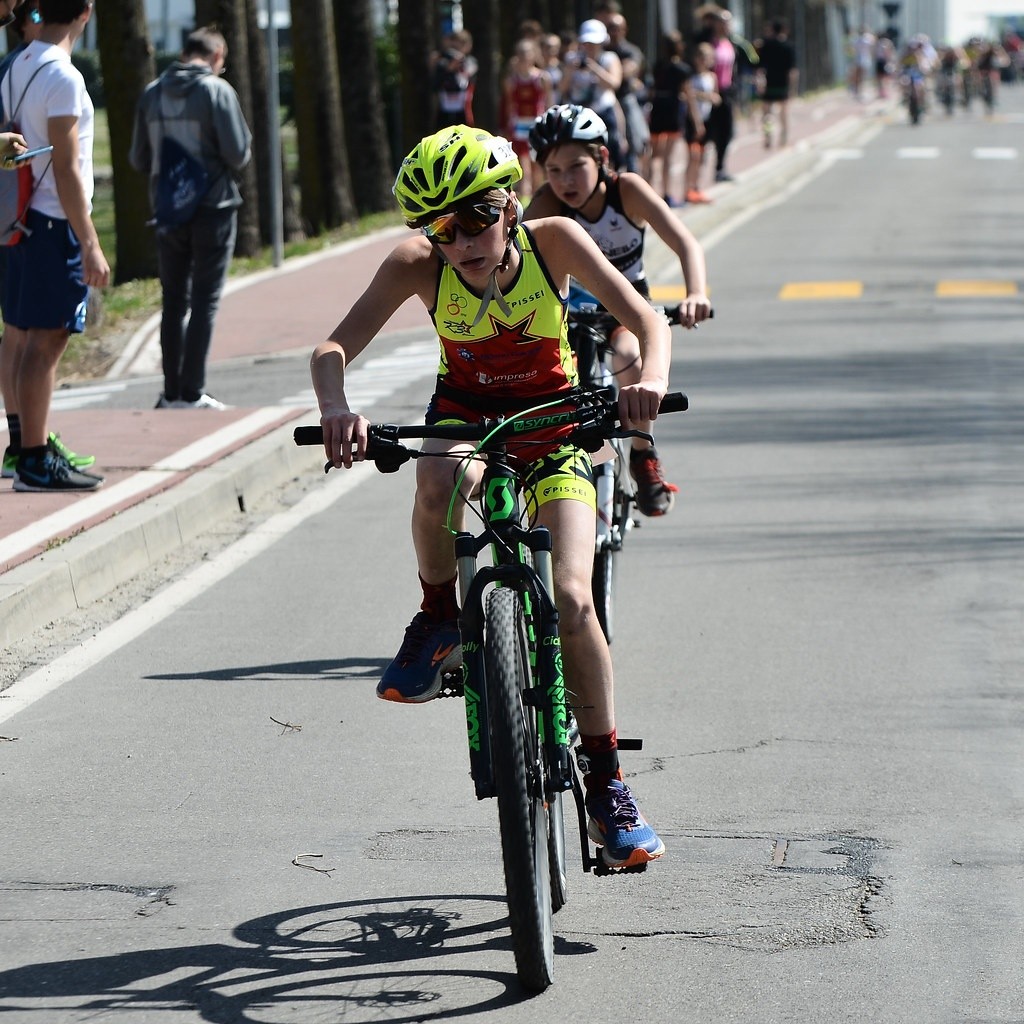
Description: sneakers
1,432,97,478
11,446,104,492
584,779,665,868
628,448,673,517
376,609,465,704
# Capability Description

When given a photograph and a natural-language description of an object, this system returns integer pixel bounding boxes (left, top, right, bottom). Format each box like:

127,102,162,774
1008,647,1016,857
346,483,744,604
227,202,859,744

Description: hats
5,0,41,28
579,19,608,45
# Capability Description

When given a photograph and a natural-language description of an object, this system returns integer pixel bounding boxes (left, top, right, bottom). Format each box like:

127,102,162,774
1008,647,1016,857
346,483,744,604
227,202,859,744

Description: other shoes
154,395,234,413
663,194,681,209
685,188,709,205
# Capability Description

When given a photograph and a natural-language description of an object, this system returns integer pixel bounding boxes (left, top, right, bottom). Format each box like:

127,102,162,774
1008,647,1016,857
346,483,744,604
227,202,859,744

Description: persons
501,0,802,208
0,0,111,491
843,25,1024,125
308,123,672,864
521,103,711,518
435,29,477,128
134,28,253,411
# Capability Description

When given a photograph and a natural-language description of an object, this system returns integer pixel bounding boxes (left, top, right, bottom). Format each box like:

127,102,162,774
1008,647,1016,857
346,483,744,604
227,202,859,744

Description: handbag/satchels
154,138,210,220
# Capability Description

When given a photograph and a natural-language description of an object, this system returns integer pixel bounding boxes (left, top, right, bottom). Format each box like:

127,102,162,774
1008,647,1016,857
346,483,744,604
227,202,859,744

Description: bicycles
551,302,717,649
291,390,689,993
898,65,999,119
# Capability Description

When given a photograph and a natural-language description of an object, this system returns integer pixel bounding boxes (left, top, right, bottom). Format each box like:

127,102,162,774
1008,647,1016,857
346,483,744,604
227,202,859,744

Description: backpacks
0,58,78,246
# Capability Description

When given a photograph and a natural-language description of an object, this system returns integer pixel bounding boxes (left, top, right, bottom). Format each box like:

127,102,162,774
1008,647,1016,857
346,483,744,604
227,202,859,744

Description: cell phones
4,145,53,162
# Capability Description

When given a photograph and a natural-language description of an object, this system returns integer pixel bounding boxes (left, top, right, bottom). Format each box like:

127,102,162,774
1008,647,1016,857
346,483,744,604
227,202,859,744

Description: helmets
393,124,522,230
527,104,608,160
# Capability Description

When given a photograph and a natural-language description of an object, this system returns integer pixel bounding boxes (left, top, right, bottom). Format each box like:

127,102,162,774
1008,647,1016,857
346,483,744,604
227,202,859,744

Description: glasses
420,185,513,244
28,9,45,24
